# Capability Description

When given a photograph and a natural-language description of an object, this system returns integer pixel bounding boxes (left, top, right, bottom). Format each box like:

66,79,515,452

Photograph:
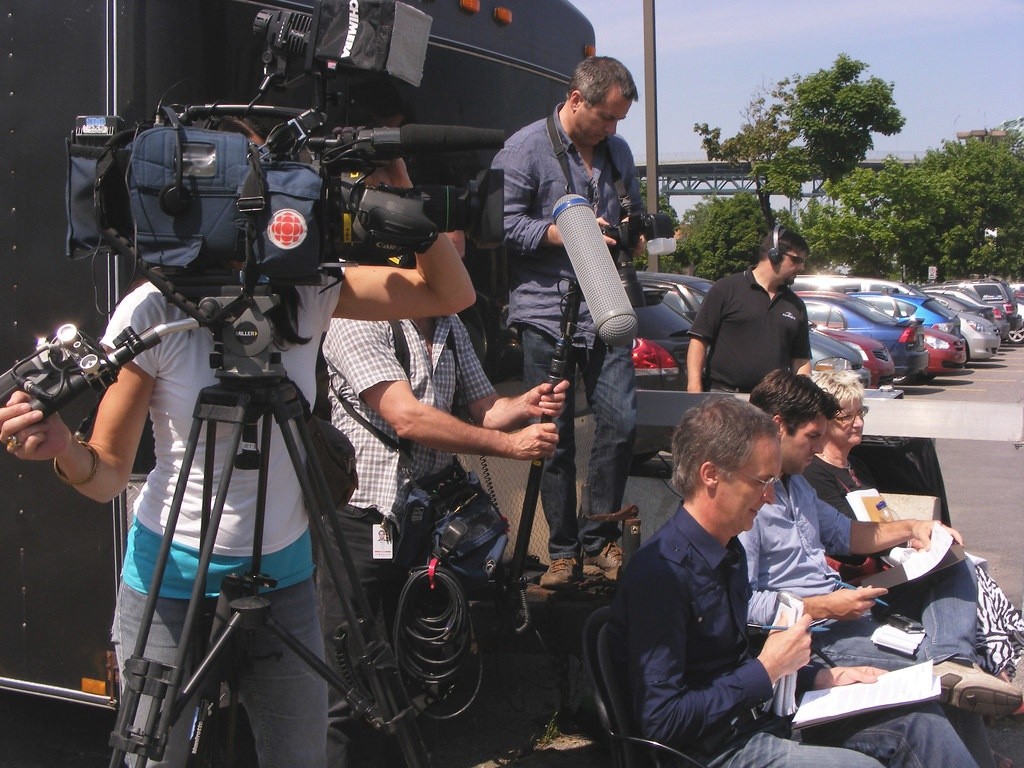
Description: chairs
582,607,708,767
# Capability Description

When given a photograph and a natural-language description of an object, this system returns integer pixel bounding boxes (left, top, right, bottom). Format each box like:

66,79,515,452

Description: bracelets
53,440,100,485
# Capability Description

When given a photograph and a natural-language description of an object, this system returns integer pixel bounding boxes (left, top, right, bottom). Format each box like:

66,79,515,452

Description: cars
924,288,1010,340
788,275,1000,360
1007,283,1024,344
847,293,961,338
810,327,895,390
799,296,930,378
620,270,872,389
794,291,966,385
924,293,1002,334
495,285,710,469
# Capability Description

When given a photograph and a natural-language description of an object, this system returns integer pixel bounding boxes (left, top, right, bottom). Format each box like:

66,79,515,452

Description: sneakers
581,540,624,579
538,556,584,588
927,657,1023,715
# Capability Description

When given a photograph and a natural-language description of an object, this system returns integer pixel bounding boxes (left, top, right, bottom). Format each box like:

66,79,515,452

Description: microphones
307,123,505,154
553,194,638,347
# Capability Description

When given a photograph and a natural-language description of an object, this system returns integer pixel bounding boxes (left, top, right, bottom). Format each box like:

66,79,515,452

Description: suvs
919,279,1024,330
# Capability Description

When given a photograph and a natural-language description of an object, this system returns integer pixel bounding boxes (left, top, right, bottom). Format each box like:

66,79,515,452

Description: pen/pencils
834,580,888,606
810,643,837,667
762,626,832,631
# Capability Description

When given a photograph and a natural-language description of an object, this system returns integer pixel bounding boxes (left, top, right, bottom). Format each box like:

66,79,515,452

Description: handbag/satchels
396,461,509,589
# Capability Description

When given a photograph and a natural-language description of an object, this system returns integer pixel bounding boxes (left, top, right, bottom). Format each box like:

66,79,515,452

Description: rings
5,434,20,450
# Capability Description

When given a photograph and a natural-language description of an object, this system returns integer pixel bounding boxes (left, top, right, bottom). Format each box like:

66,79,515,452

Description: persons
322,213,570,768
0,110,477,768
487,56,644,586
687,228,811,395
609,394,980,768
738,368,1024,718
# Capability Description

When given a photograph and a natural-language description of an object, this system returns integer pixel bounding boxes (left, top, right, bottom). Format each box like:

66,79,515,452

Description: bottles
875,500,912,548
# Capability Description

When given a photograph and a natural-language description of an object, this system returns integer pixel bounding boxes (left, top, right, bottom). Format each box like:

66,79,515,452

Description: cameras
886,614,922,634
601,213,677,258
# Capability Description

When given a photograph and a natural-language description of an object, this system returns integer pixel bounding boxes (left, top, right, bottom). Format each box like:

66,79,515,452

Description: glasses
783,251,806,265
832,406,869,423
720,462,783,491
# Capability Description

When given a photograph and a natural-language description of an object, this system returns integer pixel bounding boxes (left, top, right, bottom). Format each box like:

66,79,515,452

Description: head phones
768,225,784,265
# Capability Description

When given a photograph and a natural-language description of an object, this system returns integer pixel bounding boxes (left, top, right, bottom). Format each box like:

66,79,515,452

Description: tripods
21,284,440,768
